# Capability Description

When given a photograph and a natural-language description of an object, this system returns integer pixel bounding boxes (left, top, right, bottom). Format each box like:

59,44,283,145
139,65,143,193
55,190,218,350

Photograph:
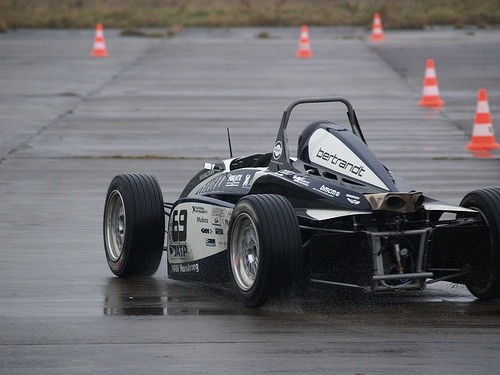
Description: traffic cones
369,13,384,41
297,25,311,60
418,60,444,107
467,89,498,150
89,24,107,57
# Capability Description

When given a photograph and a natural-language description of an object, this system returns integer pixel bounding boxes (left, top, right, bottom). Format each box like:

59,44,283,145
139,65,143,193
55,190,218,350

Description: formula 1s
102,97,500,306
